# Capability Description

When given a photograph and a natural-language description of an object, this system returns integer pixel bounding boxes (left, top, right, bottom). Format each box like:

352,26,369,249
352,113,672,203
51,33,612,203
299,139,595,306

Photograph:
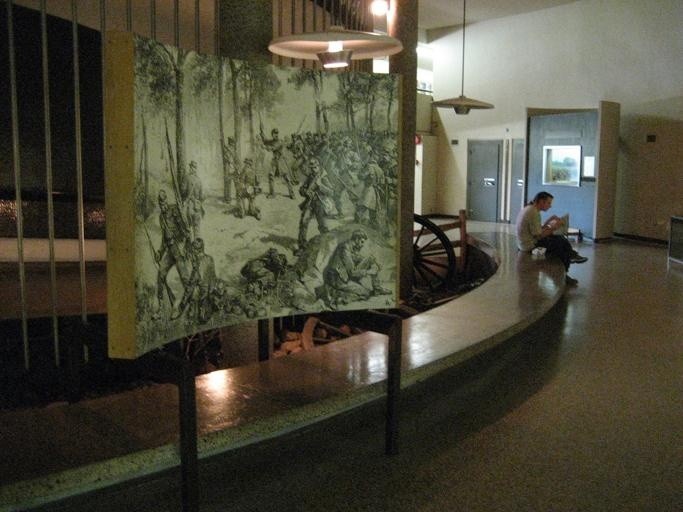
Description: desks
665,213,682,272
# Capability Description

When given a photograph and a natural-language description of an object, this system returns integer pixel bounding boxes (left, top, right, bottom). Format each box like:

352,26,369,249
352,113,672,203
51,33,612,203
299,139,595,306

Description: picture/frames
541,144,582,189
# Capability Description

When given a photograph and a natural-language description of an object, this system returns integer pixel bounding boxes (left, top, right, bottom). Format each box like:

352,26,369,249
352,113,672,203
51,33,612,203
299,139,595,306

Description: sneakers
569,253,588,265
565,275,577,286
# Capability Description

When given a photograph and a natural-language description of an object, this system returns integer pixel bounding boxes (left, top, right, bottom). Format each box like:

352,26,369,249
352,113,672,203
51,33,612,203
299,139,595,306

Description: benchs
2,227,574,510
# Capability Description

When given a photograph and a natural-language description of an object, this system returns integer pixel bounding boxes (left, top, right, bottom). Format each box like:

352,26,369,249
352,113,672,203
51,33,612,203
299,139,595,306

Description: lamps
264,0,402,73
430,2,493,120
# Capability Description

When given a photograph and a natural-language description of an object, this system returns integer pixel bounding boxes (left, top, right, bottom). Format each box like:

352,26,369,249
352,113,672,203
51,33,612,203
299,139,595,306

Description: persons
515,192,588,286
